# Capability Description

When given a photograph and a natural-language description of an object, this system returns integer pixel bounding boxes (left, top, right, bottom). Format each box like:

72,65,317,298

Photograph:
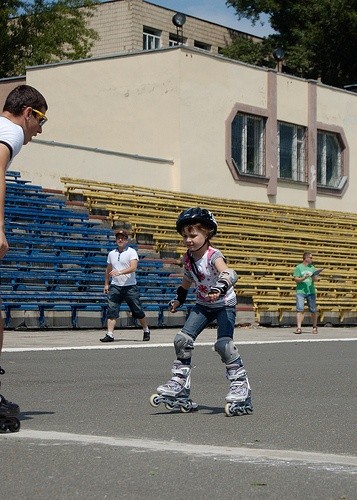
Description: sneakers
99,334,114,342
143,330,151,341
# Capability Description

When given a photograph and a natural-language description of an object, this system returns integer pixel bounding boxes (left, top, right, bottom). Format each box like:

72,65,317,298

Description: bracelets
119,271,121,275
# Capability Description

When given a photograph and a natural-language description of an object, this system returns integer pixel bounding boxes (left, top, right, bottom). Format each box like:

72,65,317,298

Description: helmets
175,207,218,235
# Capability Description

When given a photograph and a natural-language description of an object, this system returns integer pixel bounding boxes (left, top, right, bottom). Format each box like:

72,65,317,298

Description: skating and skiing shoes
0,393,21,433
224,364,254,417
149,359,199,413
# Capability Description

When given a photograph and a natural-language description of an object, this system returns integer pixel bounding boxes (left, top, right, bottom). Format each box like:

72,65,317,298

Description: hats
115,230,129,238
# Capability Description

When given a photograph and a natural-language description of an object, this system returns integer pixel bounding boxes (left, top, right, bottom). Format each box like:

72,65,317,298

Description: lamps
273,48,284,72
172,13,186,46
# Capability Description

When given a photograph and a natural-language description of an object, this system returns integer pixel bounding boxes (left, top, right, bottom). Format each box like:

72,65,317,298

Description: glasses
307,257,313,259
22,104,48,128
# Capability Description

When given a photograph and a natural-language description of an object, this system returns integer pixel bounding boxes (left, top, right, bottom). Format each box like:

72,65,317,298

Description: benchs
0,170,357,328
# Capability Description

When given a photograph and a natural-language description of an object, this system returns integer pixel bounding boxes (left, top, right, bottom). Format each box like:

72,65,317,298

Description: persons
100,229,151,342
149,206,252,416
0,84,48,432
293,252,320,334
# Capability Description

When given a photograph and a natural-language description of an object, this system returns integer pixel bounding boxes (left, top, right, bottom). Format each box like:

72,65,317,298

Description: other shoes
312,326,318,334
295,328,303,334
